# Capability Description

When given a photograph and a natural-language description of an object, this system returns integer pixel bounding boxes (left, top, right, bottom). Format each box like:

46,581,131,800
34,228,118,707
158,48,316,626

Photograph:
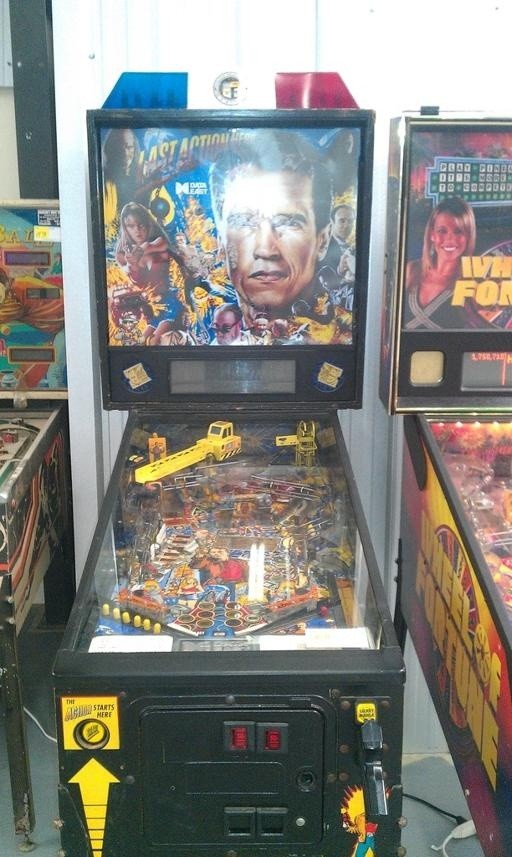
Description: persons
405,197,478,329
101,128,357,347
